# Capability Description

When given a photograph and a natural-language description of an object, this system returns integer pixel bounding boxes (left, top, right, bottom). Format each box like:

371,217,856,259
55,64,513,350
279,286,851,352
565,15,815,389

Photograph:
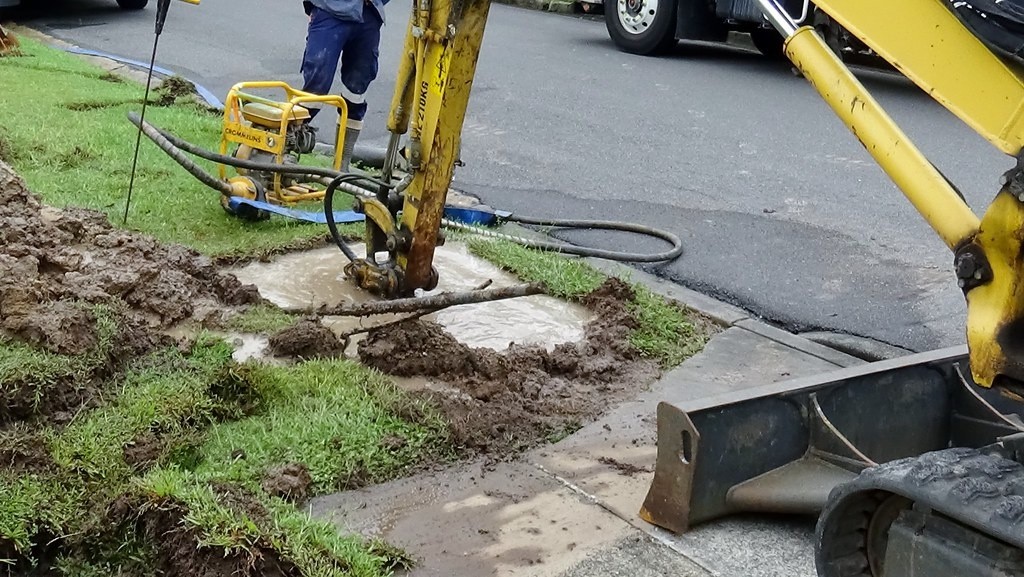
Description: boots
333,114,364,174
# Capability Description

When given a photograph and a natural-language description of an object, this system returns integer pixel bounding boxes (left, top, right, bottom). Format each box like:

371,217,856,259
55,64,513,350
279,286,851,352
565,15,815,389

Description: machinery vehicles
604,0,1024,65
325,0,1024,577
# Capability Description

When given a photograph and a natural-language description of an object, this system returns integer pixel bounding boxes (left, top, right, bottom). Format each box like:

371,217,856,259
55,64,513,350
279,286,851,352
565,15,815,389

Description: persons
300,0,391,173
